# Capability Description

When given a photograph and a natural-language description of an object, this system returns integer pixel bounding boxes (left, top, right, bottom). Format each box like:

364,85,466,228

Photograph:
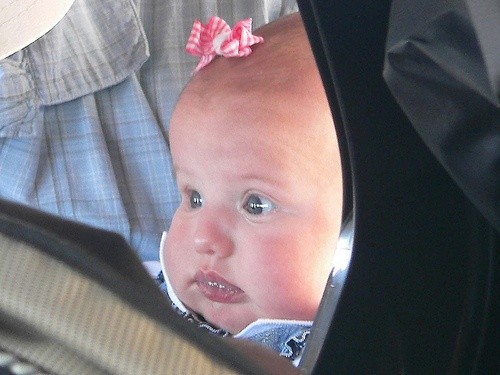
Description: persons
155,7,346,370
